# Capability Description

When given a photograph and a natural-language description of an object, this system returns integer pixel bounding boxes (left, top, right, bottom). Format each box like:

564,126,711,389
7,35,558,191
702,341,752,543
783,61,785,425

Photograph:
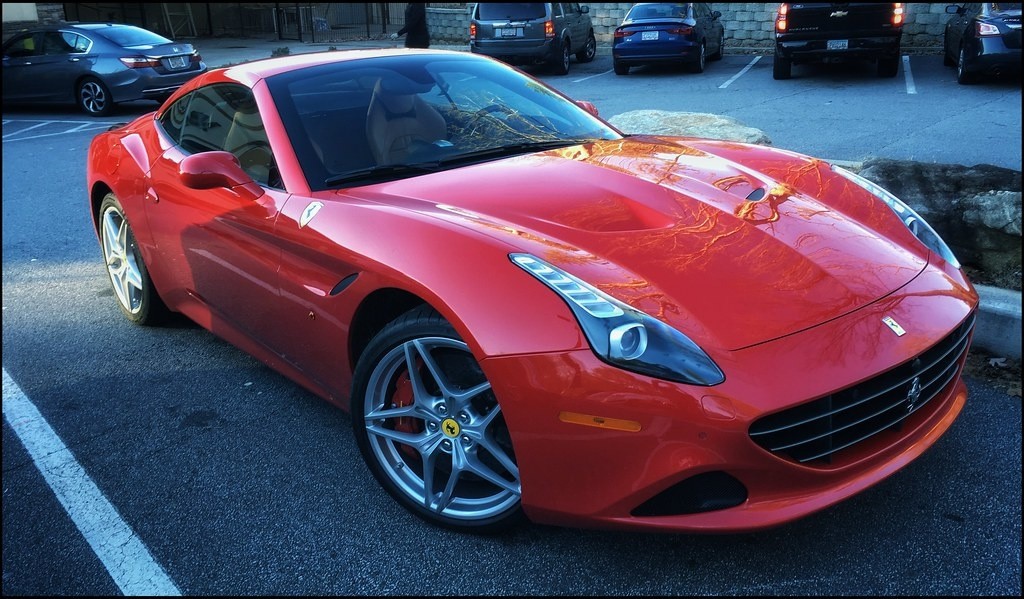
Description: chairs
222,104,321,187
363,76,446,167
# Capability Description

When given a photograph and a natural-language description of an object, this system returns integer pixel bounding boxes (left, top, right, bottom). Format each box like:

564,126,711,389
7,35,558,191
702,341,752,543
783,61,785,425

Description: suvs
773,1,904,79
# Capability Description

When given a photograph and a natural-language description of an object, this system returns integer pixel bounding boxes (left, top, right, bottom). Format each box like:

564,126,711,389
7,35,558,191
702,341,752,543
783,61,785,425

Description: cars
612,3,725,74
0,21,209,117
944,3,1024,84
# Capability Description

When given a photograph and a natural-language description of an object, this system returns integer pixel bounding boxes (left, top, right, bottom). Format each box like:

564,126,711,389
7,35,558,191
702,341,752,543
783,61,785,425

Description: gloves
391,33,398,41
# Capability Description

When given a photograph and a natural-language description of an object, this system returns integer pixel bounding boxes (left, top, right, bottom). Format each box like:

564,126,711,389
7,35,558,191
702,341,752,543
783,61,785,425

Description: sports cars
86,49,980,532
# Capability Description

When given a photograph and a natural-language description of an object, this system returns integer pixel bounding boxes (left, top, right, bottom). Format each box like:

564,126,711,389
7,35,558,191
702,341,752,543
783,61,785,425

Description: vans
470,1,597,75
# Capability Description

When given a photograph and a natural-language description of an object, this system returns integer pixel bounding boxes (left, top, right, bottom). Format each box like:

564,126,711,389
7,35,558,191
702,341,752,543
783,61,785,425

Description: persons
390,2,431,48
